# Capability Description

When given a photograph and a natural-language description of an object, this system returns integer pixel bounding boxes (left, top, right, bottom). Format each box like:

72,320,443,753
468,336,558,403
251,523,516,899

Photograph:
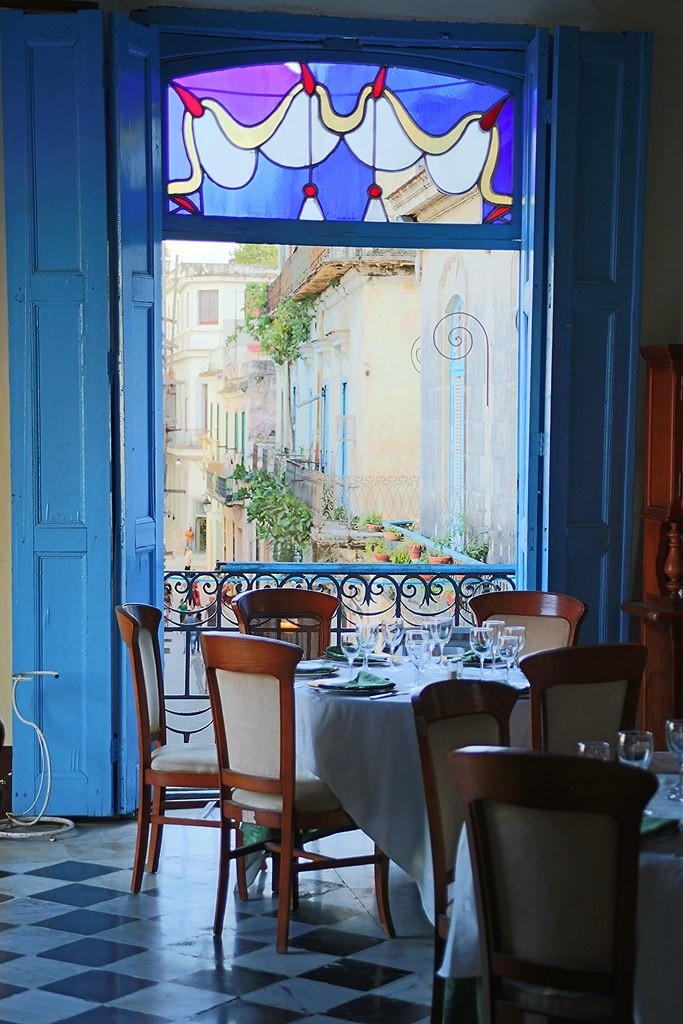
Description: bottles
457,659,464,679
447,657,453,680
452,660,458,679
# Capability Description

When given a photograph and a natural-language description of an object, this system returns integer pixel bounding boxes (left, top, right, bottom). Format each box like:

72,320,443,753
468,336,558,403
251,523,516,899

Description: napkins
324,644,389,663
294,664,339,674
322,670,397,691
641,815,681,846
450,642,508,663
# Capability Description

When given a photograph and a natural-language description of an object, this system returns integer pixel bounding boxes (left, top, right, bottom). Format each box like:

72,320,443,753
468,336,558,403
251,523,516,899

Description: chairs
115,590,661,1024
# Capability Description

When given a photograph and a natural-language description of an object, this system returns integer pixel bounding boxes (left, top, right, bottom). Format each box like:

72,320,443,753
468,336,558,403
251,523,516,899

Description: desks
236,655,533,925
436,750,683,1024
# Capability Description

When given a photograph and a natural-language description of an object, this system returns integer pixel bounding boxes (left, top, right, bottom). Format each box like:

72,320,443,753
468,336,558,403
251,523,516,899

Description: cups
577,741,610,761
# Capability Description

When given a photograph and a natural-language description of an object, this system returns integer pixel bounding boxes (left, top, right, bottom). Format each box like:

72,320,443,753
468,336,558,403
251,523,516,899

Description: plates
294,667,339,681
304,682,397,695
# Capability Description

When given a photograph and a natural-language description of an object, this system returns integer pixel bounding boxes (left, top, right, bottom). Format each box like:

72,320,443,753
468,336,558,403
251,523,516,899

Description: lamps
202,494,211,504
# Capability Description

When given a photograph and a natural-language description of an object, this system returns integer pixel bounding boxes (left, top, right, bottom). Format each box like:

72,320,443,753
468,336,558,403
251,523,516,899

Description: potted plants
362,514,491,610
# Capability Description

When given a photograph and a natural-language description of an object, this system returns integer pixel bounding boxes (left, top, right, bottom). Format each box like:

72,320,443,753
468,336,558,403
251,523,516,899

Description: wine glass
381,618,404,673
482,621,506,677
506,626,526,678
421,622,436,665
341,632,360,678
617,730,654,817
498,636,518,682
430,618,453,671
664,719,683,801
470,628,493,680
404,631,429,686
406,640,430,690
356,625,378,673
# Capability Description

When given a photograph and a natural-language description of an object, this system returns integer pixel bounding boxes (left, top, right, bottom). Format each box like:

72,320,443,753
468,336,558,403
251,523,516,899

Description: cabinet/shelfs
621,342,683,748
